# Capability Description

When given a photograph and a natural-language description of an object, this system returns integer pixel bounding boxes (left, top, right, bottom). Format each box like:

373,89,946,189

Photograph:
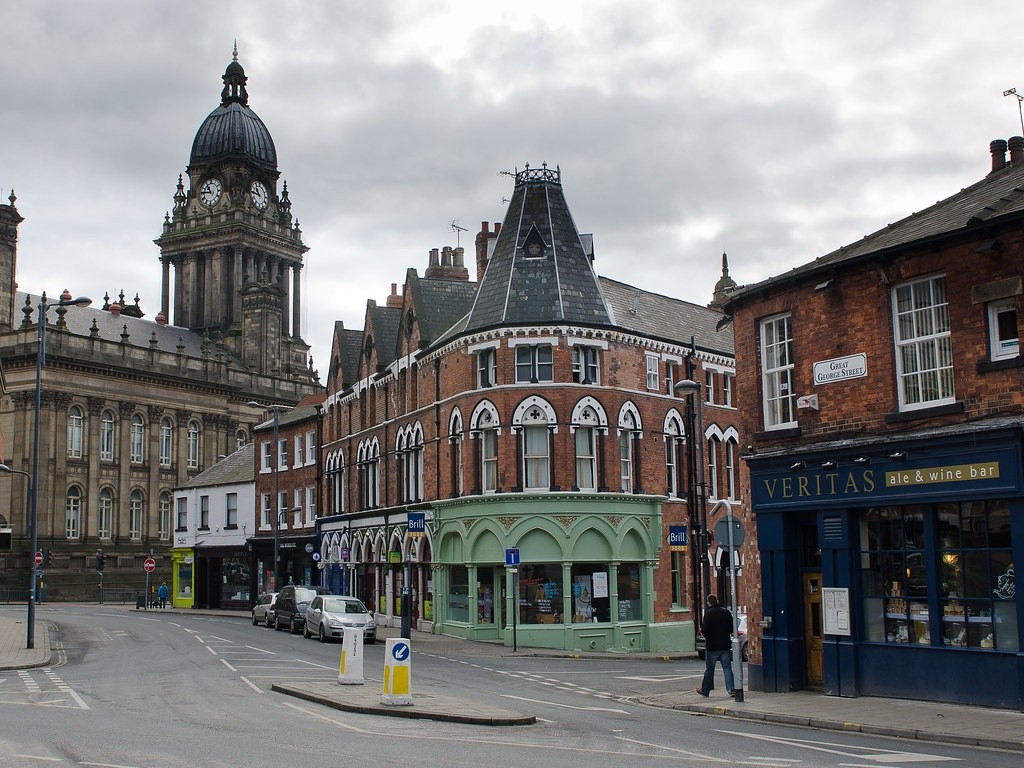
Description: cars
696,615,748,662
252,592,279,628
303,594,377,644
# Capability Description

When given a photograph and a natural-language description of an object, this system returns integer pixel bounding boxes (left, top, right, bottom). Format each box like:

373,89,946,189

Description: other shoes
730,692,735,697
696,687,709,697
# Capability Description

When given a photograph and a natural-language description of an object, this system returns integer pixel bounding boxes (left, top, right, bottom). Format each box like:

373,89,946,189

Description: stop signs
36,552,43,564
145,559,158,574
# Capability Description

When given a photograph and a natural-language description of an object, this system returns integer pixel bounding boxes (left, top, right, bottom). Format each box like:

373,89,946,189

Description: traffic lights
99,554,106,571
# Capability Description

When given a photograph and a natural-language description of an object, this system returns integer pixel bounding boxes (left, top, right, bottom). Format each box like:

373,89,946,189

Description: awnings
174,535,253,546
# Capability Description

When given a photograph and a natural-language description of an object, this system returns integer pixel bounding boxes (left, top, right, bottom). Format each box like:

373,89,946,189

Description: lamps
791,451,910,474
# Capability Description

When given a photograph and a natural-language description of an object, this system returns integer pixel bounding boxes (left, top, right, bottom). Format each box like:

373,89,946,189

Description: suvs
274,583,333,635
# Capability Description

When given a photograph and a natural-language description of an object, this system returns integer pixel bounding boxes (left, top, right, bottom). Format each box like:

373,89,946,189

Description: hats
162,582,166,585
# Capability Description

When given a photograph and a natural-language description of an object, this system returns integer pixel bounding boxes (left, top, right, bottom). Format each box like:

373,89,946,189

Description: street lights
0,297,92,649
245,400,304,593
672,378,713,615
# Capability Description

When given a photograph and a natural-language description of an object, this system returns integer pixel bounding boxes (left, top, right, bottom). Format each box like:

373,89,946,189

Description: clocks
249,180,270,213
199,178,222,207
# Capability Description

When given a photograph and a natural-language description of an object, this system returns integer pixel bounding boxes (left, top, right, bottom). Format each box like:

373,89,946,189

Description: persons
158,582,168,609
697,594,734,697
